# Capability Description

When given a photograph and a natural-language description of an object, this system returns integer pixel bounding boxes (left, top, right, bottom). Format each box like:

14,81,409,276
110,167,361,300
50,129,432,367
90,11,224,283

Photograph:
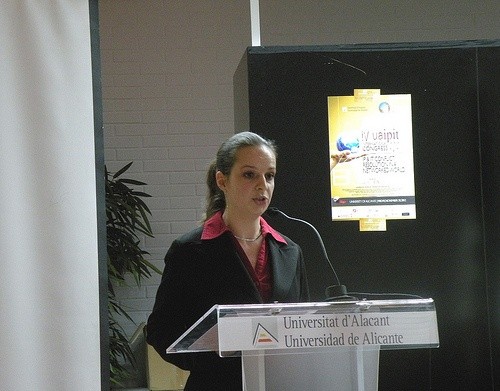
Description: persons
146,130,312,391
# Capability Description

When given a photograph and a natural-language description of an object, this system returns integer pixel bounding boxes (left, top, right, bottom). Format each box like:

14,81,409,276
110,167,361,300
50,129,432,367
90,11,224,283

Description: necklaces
230,230,263,242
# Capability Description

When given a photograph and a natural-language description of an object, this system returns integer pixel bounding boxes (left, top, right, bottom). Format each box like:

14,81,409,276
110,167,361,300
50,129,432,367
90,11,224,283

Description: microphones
268,207,358,302
